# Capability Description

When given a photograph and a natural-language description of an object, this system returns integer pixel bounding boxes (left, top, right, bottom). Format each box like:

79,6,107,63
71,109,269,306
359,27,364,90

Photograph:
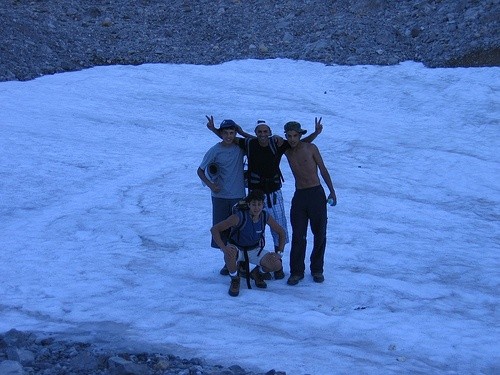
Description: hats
284,121,307,135
254,119,272,136
218,119,237,130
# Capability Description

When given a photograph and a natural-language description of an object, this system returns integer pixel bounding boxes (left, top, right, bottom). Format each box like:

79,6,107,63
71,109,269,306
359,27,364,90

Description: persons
197,113,336,285
210,188,286,296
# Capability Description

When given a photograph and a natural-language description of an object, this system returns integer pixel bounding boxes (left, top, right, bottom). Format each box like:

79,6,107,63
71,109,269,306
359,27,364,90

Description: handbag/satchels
262,172,283,208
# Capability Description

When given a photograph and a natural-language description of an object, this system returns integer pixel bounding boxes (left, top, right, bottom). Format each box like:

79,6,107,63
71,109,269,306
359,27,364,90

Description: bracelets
278,250,283,253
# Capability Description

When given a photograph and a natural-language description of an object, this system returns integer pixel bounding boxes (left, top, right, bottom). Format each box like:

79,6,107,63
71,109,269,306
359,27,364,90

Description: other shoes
249,265,267,288
274,269,284,280
286,274,305,285
228,271,241,297
220,264,229,275
311,272,325,283
